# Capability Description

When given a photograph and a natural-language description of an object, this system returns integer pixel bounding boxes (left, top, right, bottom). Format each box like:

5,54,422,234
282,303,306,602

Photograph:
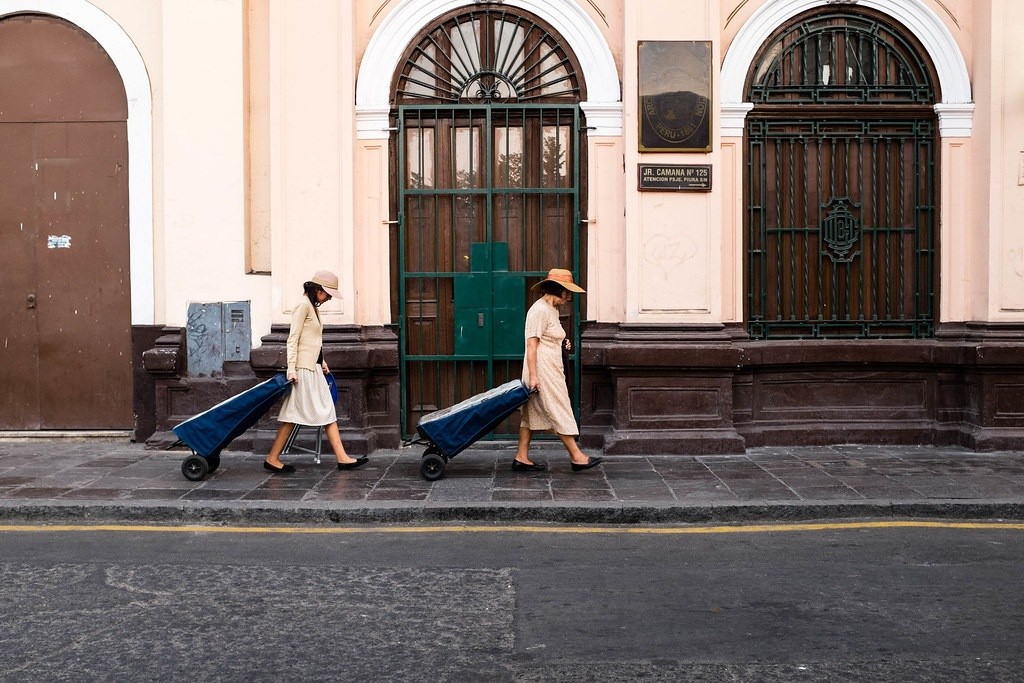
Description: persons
512,269,601,470
263,270,369,473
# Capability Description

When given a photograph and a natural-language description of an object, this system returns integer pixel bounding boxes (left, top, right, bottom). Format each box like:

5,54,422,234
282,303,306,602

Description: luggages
167,374,295,481
402,379,537,480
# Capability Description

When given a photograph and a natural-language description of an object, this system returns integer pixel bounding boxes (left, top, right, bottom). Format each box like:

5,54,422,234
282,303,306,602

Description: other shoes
263,460,296,473
512,459,545,472
338,458,369,471
571,456,602,472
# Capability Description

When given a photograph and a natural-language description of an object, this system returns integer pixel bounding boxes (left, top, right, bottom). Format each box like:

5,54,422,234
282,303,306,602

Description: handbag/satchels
324,370,338,404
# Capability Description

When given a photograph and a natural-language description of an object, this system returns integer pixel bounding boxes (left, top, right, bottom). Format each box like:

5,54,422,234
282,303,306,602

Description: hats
531,269,586,294
312,270,343,299
